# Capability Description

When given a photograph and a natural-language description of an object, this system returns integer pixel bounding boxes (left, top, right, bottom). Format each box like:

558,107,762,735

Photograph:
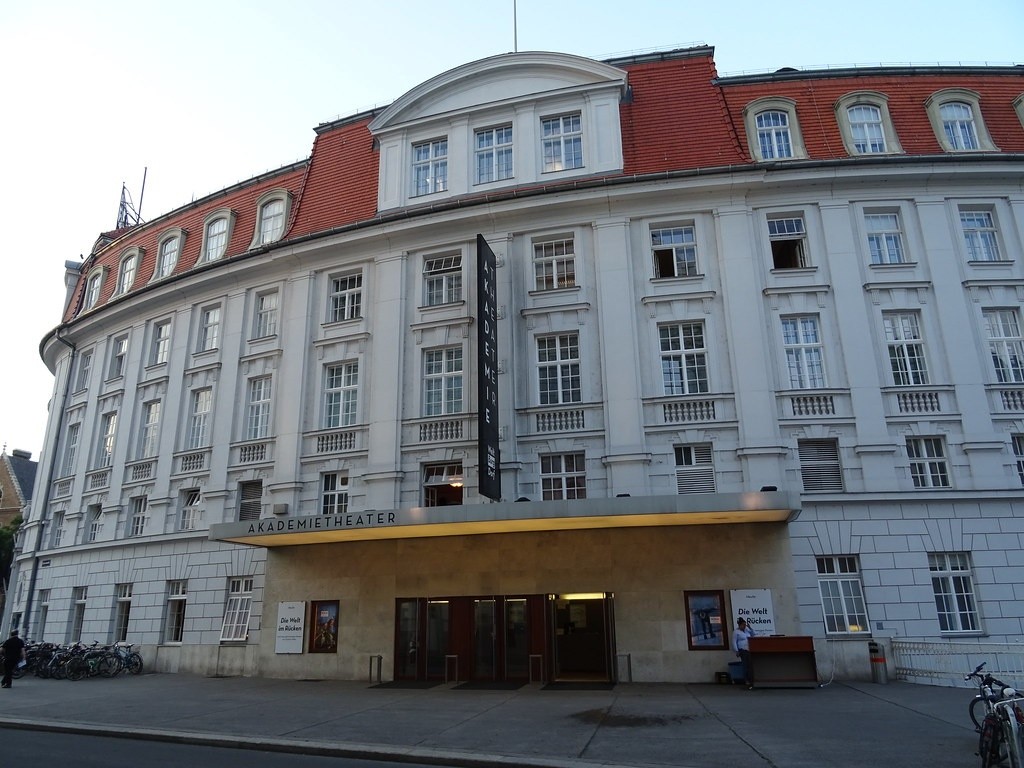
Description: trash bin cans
868,642,887,684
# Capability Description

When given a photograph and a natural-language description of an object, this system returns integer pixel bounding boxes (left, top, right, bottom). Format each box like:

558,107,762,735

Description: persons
732,620,756,690
0,629,26,688
698,609,716,639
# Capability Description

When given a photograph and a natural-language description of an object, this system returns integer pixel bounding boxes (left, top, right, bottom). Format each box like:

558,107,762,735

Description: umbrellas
693,607,719,615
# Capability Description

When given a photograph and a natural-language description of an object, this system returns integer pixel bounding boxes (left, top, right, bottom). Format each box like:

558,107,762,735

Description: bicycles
964,661,1024,768
0,637,144,681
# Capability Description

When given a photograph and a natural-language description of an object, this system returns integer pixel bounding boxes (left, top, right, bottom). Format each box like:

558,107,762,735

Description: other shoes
2,684,11,688
745,683,753,690
1,681,5,686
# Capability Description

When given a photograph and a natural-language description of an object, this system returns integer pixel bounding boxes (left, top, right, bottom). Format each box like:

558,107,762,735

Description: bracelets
23,657,25,658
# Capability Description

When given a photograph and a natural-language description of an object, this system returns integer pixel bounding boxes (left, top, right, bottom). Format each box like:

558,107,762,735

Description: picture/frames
308,599,340,654
683,589,731,652
